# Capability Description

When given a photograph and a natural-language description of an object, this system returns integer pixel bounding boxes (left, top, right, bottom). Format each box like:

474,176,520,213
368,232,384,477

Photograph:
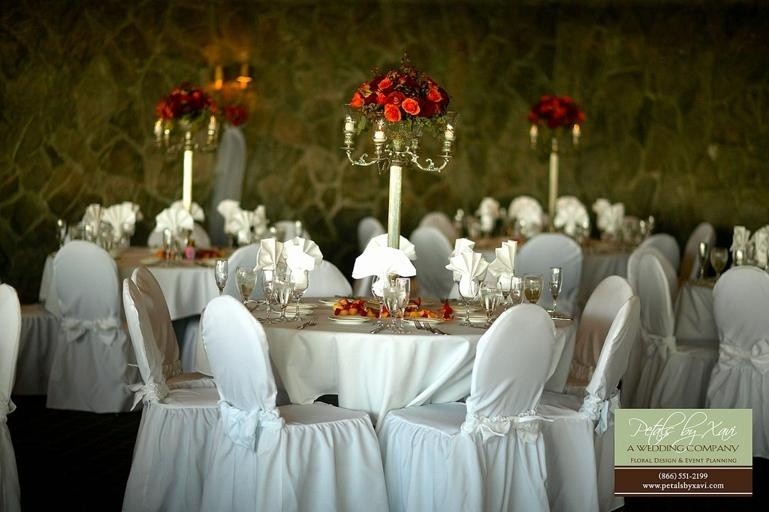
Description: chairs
0,194,768,511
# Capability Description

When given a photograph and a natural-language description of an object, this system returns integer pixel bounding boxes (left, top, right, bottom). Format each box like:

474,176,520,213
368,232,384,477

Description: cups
51,211,257,265
696,238,749,284
465,209,655,248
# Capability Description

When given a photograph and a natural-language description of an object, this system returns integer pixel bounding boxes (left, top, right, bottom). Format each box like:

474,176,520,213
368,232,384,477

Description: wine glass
457,266,563,327
382,278,411,334
214,258,312,323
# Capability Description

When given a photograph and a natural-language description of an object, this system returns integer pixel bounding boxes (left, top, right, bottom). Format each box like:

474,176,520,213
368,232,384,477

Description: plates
319,298,337,306
328,314,370,325
404,318,445,328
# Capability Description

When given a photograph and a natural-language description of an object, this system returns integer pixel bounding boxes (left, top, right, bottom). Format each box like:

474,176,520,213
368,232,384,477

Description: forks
413,318,448,336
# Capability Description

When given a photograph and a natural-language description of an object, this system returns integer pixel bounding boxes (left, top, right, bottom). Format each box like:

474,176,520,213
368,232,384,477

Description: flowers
152,82,250,128
345,48,456,124
524,93,590,129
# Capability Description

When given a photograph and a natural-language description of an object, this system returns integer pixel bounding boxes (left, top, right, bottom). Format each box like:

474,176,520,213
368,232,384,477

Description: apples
334,297,453,319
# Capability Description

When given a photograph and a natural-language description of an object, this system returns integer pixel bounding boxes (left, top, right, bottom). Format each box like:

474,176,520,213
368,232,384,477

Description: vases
527,123,585,235
151,114,223,259
340,114,462,316
